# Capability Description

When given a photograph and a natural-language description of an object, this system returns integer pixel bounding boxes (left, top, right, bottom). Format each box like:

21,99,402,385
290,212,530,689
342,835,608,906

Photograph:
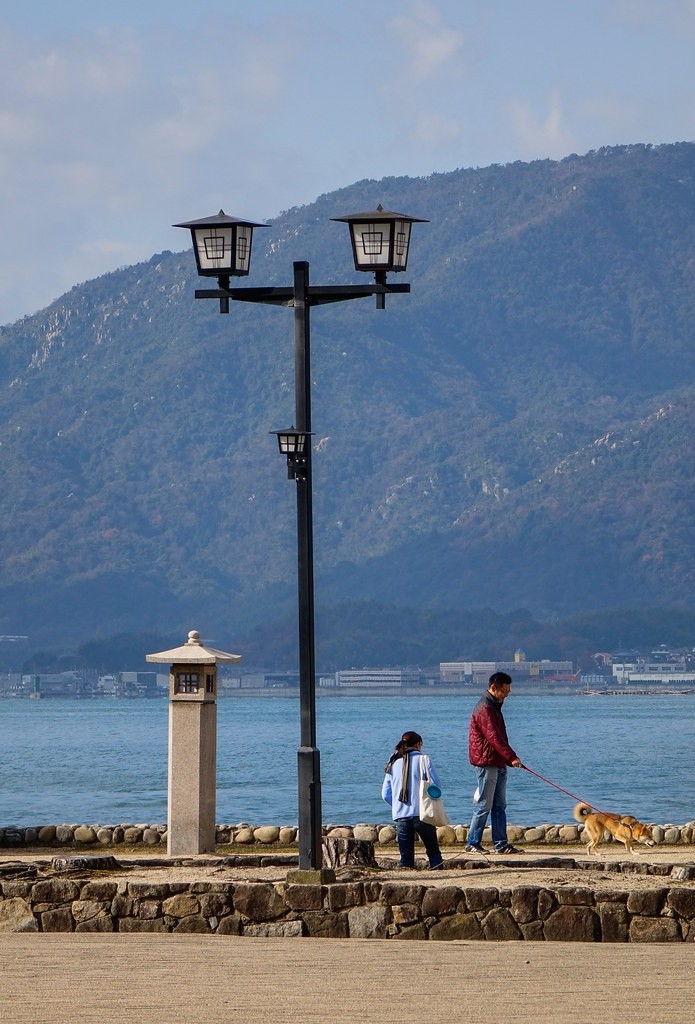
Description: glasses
498,688,511,695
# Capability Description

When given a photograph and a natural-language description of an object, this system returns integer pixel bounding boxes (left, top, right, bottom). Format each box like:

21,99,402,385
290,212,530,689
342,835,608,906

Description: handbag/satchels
419,755,449,827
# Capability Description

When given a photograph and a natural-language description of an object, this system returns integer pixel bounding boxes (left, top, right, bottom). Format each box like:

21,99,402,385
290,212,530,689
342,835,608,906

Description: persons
465,671,523,858
382,732,445,870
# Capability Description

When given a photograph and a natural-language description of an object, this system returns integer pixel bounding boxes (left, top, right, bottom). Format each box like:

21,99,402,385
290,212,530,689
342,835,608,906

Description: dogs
573,802,657,855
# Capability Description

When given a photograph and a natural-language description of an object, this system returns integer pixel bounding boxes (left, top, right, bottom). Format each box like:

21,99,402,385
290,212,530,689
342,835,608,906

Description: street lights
173,204,430,884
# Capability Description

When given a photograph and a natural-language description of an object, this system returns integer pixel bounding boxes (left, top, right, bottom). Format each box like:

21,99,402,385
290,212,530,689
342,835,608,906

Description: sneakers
495,844,525,855
466,845,490,855
